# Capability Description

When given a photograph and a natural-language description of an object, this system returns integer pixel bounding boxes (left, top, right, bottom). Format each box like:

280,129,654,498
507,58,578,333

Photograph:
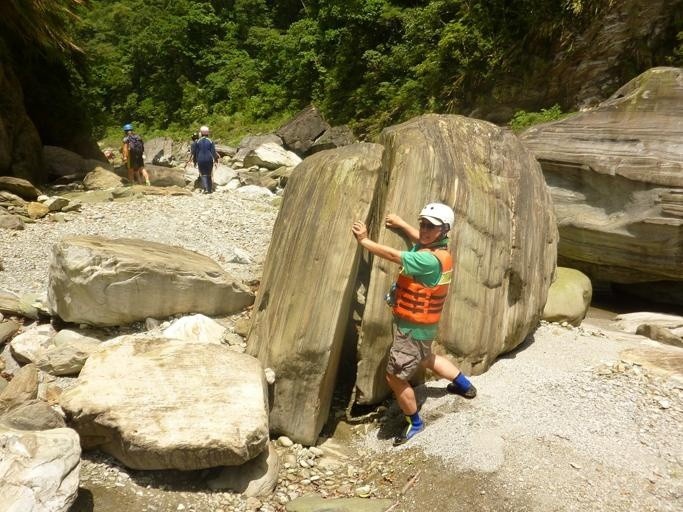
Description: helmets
124,124,134,131
200,126,209,135
418,202,455,231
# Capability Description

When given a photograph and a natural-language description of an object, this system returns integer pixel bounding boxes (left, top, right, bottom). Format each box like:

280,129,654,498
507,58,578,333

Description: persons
191,132,220,186
352,202,477,445
104,124,150,186
193,126,219,193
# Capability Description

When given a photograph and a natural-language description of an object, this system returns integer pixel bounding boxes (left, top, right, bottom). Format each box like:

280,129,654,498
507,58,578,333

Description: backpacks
127,134,144,160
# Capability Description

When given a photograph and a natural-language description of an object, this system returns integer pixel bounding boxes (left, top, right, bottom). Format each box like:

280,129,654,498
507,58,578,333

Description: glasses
419,222,443,230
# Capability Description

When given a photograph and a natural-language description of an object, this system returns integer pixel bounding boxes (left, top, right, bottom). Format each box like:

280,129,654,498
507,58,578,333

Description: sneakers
447,372,477,398
393,411,425,446
